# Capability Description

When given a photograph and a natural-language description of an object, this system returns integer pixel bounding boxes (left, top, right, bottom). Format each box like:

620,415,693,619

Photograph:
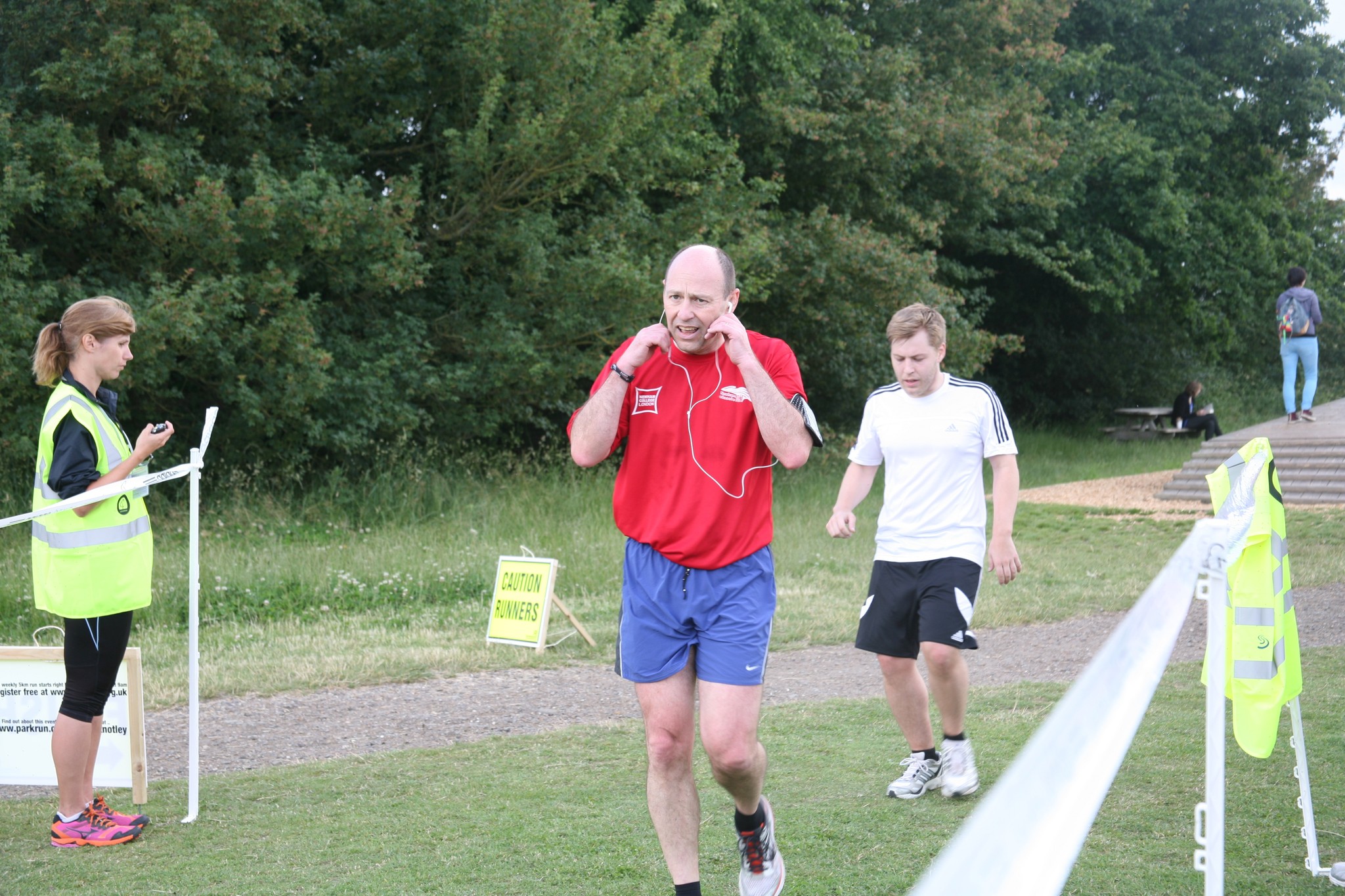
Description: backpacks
1276,297,1309,343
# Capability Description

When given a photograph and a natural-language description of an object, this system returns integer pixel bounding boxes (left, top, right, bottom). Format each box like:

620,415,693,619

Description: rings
726,322,728,325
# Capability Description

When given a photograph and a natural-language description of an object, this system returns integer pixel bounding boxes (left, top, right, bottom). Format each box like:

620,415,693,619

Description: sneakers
89,793,150,831
735,795,785,896
886,751,945,798
940,739,979,798
50,802,143,848
1287,412,1302,424
1301,409,1316,422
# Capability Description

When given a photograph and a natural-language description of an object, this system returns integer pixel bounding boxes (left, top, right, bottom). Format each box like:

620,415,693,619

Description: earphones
728,302,733,313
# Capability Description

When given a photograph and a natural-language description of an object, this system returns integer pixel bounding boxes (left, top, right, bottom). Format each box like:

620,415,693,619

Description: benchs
1164,428,1190,434
1101,424,1149,432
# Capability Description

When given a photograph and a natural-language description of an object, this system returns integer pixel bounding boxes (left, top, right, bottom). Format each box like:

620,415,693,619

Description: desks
1114,406,1173,443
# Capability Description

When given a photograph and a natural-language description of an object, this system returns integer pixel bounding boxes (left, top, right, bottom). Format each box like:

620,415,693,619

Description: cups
1176,417,1183,429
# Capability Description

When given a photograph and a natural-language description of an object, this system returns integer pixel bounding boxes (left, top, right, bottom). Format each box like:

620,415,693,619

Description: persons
567,241,824,896
32,295,175,848
826,302,1022,799
1170,380,1222,442
1276,268,1322,423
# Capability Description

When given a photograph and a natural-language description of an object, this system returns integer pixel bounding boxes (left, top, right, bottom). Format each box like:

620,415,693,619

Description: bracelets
610,363,635,383
